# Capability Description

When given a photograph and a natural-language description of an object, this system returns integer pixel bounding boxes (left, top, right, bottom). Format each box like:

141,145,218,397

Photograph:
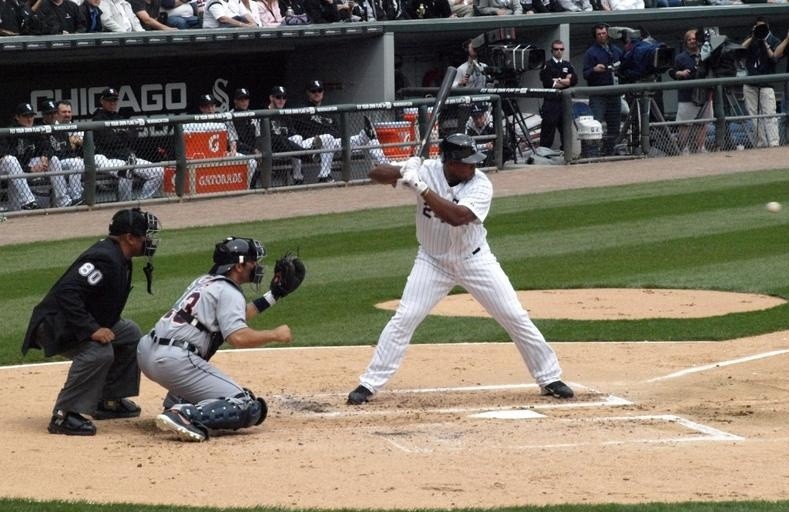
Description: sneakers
308,134,322,164
48,410,96,435
363,115,378,140
319,175,335,182
348,385,372,404
540,380,574,399
117,153,136,180
93,397,141,420
21,200,41,209
71,198,83,206
155,410,206,442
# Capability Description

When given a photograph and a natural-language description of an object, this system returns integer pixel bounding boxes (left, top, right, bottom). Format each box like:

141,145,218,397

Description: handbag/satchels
283,13,313,24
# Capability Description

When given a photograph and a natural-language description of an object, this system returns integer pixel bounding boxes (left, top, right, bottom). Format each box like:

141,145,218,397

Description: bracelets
253,297,270,313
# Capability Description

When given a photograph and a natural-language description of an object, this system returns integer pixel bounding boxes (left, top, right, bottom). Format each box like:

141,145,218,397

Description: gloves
399,156,421,177
401,170,428,195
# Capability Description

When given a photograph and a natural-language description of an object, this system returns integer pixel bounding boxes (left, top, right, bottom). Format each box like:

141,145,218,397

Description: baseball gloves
271,257,306,298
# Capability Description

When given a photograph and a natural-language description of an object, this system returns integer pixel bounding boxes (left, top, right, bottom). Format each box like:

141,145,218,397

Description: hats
102,88,119,100
41,100,58,115
234,87,250,99
439,133,488,165
199,94,217,107
308,79,323,92
16,103,37,116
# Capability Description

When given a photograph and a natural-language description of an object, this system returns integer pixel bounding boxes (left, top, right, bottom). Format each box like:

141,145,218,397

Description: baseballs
767,203,780,212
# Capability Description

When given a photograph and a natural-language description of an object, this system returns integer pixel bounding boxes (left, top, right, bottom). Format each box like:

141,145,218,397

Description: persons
285,0,594,24
344,132,576,406
200,80,376,186
451,44,496,162
21,206,162,437
601,1,646,11
134,235,306,443
0,1,285,38
0,87,165,208
540,18,788,157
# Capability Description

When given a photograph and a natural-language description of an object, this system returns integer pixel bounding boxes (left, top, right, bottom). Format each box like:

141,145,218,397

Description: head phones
460,41,468,57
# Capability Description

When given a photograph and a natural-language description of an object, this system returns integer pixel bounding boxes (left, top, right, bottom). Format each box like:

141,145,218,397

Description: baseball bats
418,66,456,157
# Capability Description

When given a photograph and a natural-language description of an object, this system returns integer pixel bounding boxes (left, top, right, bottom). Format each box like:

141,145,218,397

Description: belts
151,330,202,356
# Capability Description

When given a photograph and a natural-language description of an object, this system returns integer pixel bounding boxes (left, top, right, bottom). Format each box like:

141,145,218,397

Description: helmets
208,236,267,284
108,208,163,257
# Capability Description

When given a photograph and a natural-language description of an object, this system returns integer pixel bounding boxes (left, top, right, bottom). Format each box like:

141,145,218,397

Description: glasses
273,95,287,99
308,88,323,93
551,47,564,51
270,85,287,97
